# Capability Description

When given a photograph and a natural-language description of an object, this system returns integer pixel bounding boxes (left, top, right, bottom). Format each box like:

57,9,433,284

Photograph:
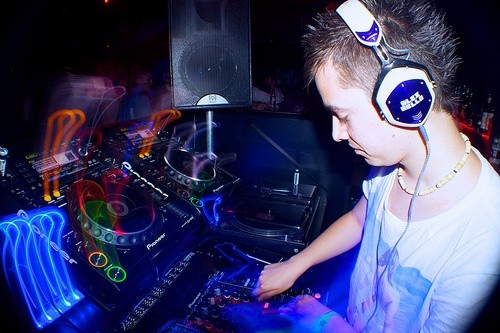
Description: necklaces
397,131,471,196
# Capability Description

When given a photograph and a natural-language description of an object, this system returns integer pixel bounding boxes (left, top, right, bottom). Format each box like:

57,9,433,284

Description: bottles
446,84,476,126
268,89,277,111
478,93,495,134
489,133,500,165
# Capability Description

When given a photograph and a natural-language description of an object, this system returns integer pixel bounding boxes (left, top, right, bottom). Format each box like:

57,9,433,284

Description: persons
84,62,285,124
251,0,500,333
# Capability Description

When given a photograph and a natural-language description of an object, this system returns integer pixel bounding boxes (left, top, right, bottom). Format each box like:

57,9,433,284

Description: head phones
336,0,437,128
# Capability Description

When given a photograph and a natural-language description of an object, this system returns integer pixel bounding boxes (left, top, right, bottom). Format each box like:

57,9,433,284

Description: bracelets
315,310,344,333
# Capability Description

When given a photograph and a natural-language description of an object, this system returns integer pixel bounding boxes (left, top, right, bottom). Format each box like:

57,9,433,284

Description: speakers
167,0,253,111
